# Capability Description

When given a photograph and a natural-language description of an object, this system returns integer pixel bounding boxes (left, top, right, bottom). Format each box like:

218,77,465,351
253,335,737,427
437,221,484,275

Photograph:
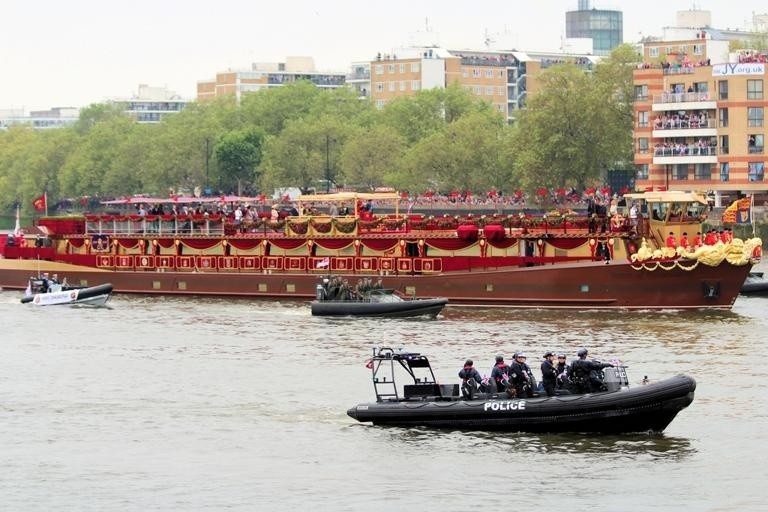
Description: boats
312,284,449,321
740,271,768,297
345,346,695,436
20,277,114,308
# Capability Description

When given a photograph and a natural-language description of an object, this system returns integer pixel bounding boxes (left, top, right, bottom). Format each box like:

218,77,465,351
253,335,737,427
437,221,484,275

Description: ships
0,190,763,312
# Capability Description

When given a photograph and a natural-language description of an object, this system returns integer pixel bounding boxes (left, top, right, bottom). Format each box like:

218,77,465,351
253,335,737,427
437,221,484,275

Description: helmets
558,354,567,359
543,351,554,358
512,353,526,360
466,359,473,365
496,356,503,362
578,350,587,356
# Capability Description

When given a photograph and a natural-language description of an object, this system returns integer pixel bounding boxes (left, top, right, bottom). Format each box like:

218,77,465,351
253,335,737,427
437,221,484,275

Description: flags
317,257,329,268
722,194,753,227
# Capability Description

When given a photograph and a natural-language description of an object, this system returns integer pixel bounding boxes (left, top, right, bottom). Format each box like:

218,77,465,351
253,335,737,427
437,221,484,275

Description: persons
375,51,592,71
583,193,639,236
666,227,734,256
320,276,385,304
329,199,373,216
136,202,280,233
36,271,71,294
458,347,615,402
291,202,317,215
50,193,113,211
7,234,16,246
627,49,768,157
35,234,43,248
400,185,629,206
